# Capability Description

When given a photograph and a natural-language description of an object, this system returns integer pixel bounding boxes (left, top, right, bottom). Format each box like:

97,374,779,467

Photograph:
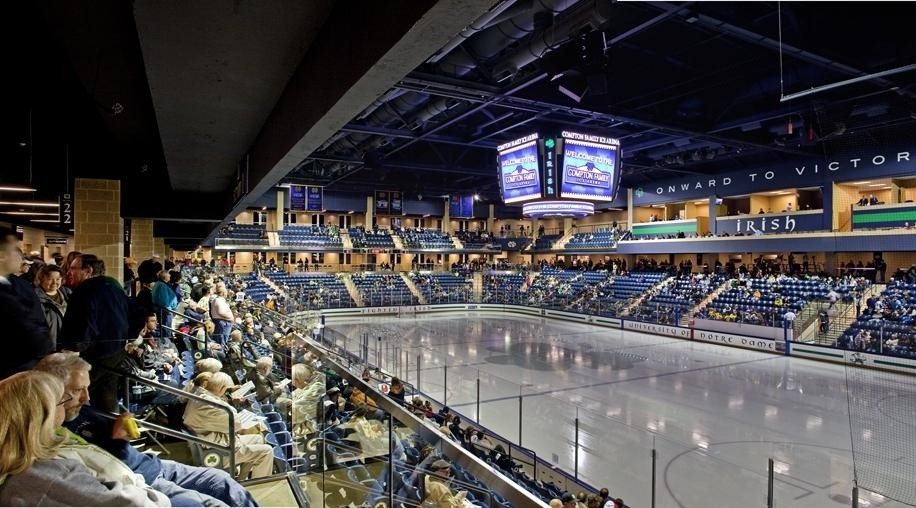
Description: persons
351,223,390,252
279,280,339,307
499,222,547,238
297,345,390,465
367,432,474,507
361,259,419,307
455,229,493,244
580,259,916,358
260,229,269,240
123,250,274,481
290,364,326,460
394,225,456,248
572,220,621,242
550,488,624,508
1,227,133,431
857,194,879,205
650,214,679,222
411,255,593,309
38,352,258,508
311,220,339,242
1,371,171,507
269,255,319,272
360,368,516,475
728,202,810,215
628,231,752,241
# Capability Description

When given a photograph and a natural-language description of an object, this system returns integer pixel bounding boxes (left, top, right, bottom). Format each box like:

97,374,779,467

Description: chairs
629,273,916,359
117,224,628,508
